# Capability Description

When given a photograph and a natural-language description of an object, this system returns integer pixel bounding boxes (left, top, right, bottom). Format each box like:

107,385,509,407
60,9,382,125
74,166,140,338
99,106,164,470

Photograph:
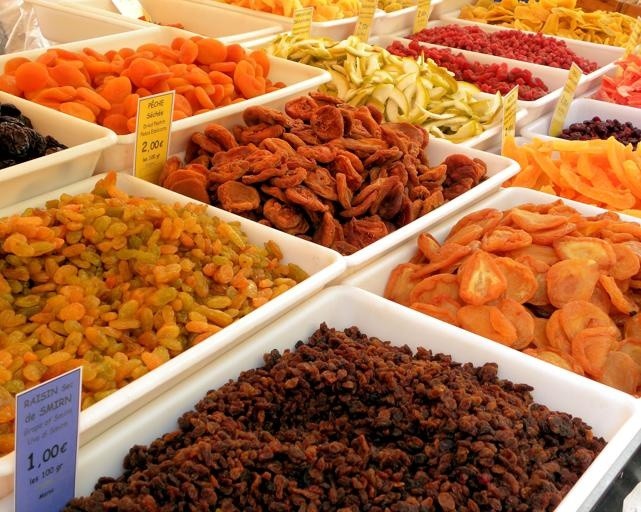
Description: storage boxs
119,133,519,265
0,169,345,475
2,2,641,221
344,187,641,442
0,287,640,512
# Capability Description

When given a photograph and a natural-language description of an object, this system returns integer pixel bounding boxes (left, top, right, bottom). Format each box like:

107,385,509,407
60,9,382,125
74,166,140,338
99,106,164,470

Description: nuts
0,0,641,512
